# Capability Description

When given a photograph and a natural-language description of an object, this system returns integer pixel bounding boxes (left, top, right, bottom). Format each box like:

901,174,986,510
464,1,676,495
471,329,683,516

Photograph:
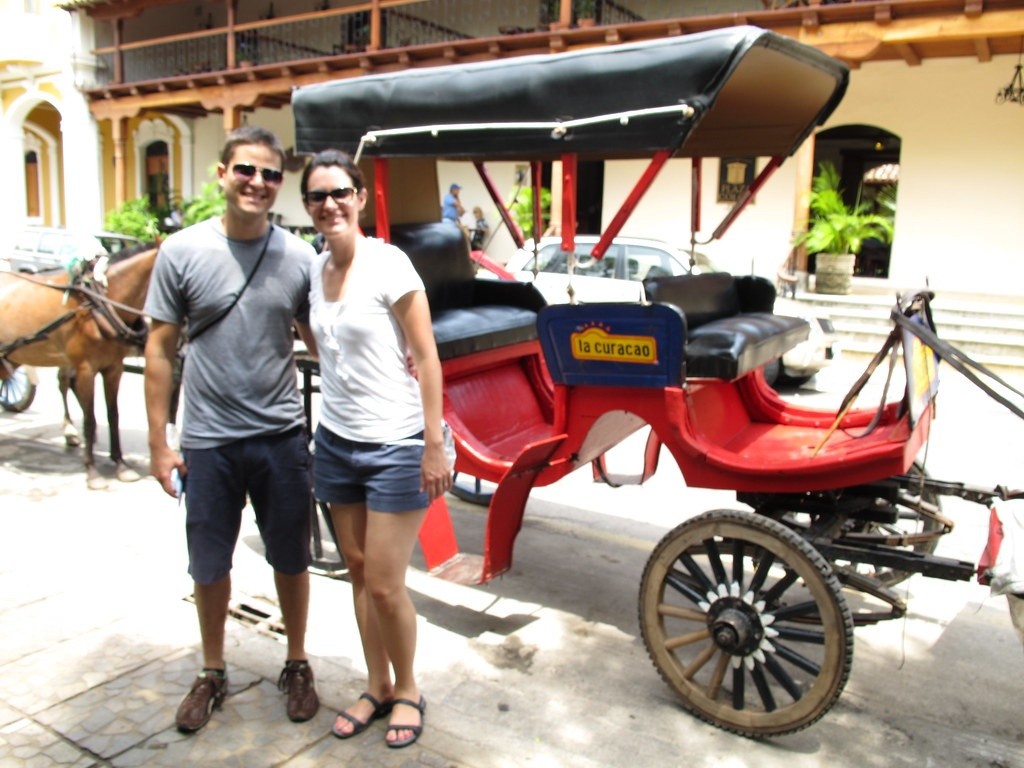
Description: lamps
994,61,1024,104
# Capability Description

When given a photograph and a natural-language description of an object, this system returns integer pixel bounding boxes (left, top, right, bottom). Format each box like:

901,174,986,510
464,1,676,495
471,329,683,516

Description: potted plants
576,0,597,27
794,161,895,296
237,49,255,67
548,1,560,30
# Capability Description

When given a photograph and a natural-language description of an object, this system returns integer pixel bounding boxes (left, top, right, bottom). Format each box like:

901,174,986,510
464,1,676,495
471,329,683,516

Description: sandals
385,694,425,747
332,693,394,739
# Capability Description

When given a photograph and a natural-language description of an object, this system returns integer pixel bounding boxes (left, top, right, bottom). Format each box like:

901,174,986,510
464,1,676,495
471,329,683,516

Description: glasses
303,187,358,206
228,164,284,185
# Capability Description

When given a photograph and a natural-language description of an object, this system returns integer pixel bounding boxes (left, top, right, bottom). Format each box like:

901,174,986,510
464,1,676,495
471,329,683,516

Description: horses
0,233,192,490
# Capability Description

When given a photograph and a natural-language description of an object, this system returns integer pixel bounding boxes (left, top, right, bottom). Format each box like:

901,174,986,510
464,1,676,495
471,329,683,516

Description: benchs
311,216,554,376
642,273,810,379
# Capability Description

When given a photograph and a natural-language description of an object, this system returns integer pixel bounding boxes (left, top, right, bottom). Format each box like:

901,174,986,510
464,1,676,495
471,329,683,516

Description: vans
9,227,146,281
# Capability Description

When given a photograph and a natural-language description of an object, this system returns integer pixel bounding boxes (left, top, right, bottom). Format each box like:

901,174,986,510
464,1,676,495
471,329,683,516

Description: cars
499,234,828,387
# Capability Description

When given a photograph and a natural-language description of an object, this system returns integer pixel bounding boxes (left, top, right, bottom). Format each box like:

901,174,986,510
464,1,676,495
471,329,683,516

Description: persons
141,125,456,747
443,183,489,250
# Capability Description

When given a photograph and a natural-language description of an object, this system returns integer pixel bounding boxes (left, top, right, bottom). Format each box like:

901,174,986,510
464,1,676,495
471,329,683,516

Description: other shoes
279,664,319,722
175,671,227,731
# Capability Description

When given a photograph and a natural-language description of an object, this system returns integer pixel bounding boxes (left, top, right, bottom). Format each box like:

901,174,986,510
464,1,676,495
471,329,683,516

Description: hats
451,184,461,190
